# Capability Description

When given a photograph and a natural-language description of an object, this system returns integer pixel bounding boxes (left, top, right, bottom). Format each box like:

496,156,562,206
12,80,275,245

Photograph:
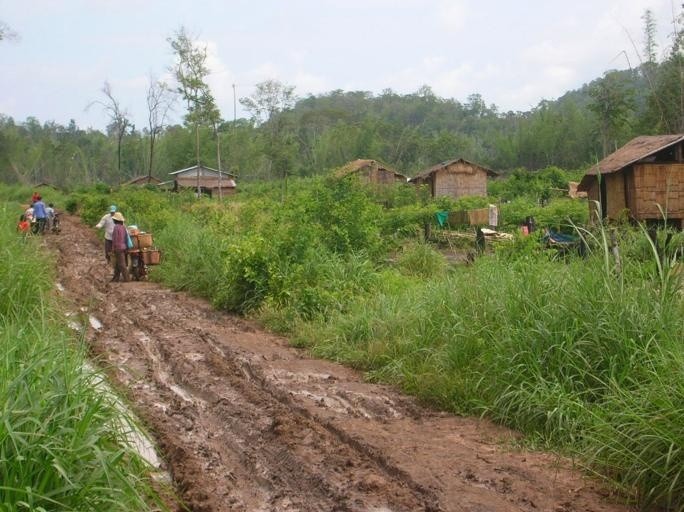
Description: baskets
130,233,161,265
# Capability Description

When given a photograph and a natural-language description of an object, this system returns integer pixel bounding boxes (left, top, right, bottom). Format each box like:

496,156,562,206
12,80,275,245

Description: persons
44,203,54,232
32,196,48,234
24,204,36,227
31,191,39,203
16,215,28,232
109,213,132,282
94,206,117,260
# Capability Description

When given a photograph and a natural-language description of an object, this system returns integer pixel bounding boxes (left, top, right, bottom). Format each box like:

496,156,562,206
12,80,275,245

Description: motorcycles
128,251,147,280
50,212,63,235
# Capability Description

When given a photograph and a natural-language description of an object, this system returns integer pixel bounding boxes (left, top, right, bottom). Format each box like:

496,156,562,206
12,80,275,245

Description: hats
110,205,125,222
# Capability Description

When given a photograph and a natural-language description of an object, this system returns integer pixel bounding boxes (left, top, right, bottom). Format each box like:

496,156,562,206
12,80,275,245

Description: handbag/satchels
126,228,134,249
